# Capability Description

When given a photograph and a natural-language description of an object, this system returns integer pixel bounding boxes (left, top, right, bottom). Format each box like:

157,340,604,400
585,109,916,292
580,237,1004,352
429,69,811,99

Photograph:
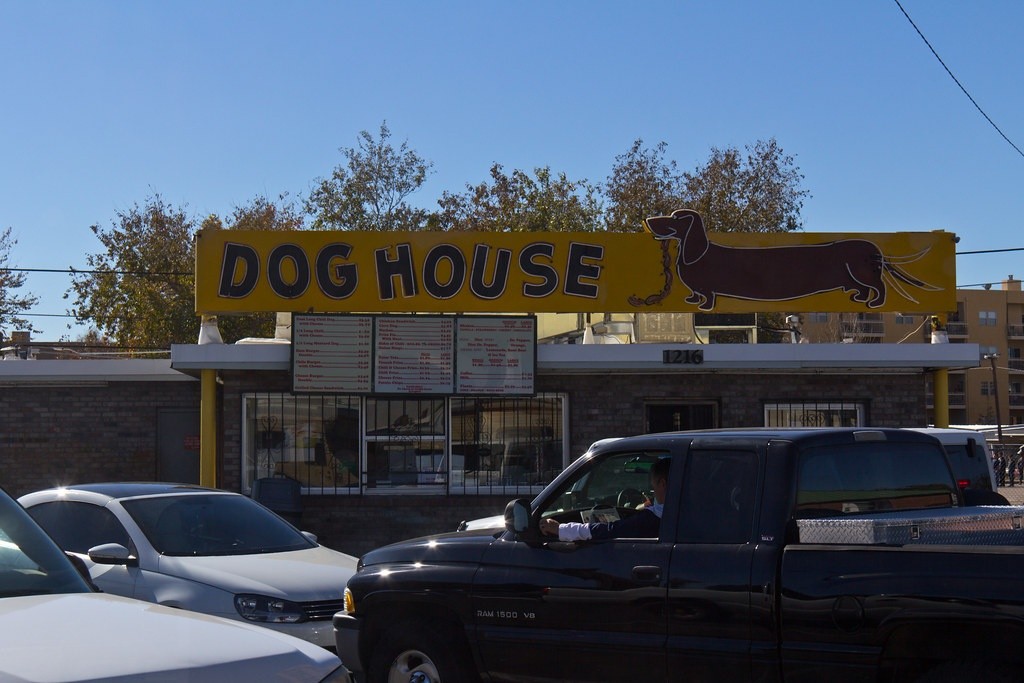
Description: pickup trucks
331,425,1023,683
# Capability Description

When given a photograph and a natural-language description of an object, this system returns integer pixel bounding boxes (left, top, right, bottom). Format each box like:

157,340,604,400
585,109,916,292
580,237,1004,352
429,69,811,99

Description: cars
0,490,355,683
1,481,361,656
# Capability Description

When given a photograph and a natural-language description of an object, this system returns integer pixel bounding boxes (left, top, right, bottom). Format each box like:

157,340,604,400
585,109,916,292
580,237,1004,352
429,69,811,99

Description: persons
538,457,671,542
992,451,1024,487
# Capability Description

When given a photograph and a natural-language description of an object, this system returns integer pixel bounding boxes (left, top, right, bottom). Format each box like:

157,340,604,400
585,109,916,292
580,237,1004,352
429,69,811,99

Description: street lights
985,354,1003,444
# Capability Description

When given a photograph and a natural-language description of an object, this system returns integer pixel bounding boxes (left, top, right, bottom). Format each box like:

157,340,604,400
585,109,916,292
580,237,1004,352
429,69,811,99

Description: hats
1017,445,1024,454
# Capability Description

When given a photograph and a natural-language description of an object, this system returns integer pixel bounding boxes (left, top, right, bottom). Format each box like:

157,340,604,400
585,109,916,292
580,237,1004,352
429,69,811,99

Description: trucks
433,439,562,487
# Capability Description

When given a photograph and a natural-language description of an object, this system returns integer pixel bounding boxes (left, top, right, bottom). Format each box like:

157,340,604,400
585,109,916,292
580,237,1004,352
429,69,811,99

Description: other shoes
1019,481,1022,485
1008,485,1014,487
999,484,1006,487
